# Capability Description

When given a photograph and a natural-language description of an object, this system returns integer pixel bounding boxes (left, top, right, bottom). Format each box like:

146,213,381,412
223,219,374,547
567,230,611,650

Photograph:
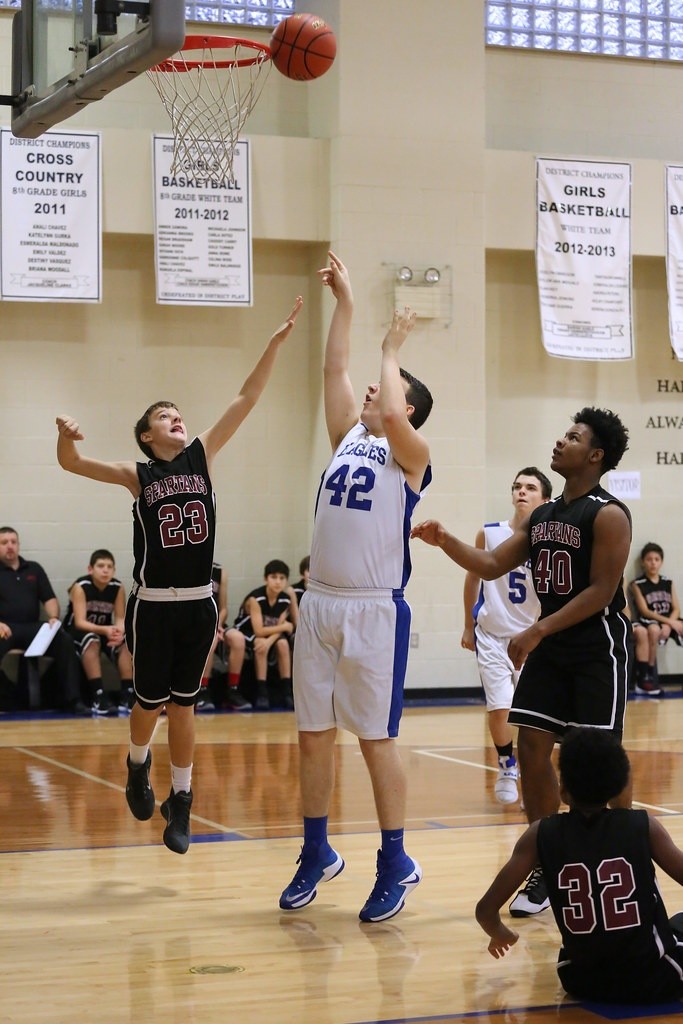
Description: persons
461,466,553,805
285,554,311,645
195,561,253,709
57,296,303,853
630,542,683,694
0,526,68,713
233,560,296,709
410,405,633,917
62,549,135,714
622,575,665,696
280,251,433,923
475,726,683,1002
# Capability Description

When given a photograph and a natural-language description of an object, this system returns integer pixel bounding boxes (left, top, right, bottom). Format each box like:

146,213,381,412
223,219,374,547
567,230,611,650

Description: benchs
0,649,278,707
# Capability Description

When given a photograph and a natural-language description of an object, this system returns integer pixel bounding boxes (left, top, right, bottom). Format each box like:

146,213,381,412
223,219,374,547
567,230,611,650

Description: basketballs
269,13,338,82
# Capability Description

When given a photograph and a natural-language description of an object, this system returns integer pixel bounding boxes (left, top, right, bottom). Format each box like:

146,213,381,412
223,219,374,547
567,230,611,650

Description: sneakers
160,785,192,854
634,672,665,697
494,754,518,805
126,749,156,820
90,688,119,714
220,686,252,711
509,862,550,917
359,847,423,922
279,842,345,909
118,688,136,713
194,686,216,710
256,686,271,711
